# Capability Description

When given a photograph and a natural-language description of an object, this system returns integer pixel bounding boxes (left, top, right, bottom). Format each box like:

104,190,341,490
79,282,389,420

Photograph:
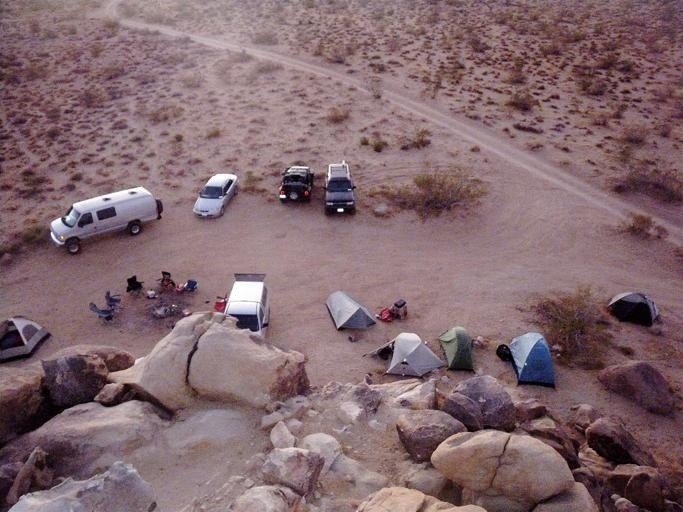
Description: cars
193,173,239,218
278,164,312,204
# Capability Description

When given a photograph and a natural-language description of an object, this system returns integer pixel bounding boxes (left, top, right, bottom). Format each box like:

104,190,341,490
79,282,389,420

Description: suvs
50,185,163,254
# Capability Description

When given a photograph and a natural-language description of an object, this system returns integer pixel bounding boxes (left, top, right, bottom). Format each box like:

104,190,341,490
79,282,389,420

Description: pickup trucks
323,162,357,214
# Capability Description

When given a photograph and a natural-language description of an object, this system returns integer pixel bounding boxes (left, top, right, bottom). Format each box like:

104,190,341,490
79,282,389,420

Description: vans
224,281,271,337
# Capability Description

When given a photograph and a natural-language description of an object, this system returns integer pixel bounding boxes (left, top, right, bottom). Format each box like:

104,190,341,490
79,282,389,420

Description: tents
325,289,379,334
437,326,476,375
369,329,446,379
506,331,556,391
605,287,665,331
